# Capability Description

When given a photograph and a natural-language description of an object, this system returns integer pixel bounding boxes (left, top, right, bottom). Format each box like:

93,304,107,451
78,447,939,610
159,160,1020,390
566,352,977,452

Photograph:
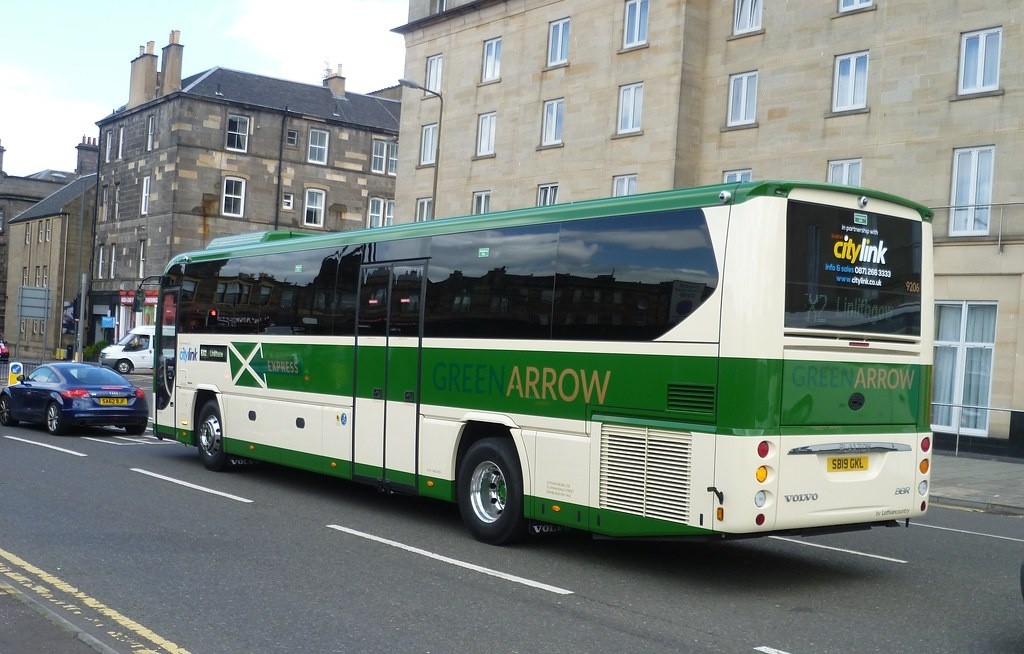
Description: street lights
50,172,86,362
398,79,444,219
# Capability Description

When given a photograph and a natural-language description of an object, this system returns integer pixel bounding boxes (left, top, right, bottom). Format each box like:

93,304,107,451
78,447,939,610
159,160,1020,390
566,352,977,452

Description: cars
0,334,10,361
0,363,149,435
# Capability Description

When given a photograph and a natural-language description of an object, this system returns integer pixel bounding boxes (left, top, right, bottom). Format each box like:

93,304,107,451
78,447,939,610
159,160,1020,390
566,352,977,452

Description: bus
130,179,937,546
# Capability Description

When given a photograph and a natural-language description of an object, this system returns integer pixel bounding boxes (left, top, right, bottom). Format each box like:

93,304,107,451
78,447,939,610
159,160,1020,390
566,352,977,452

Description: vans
102,326,177,373
